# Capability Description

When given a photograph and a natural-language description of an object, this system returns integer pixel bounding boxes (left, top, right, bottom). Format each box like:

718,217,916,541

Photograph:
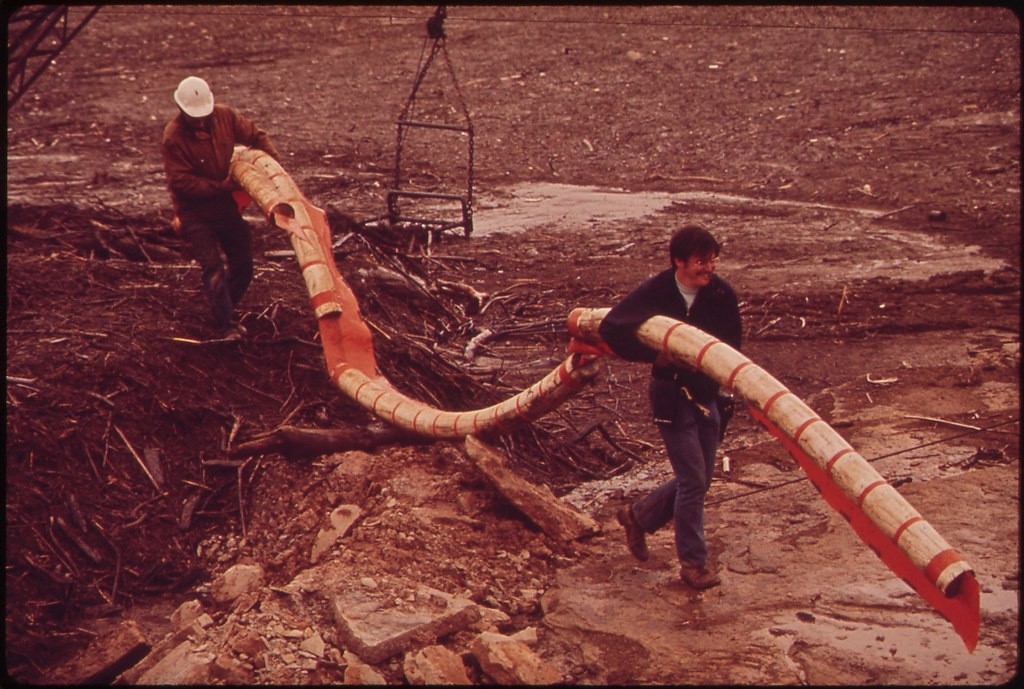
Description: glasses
685,256,719,269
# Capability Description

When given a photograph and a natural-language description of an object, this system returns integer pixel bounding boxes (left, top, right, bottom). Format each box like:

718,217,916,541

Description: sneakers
617,505,648,562
680,566,720,589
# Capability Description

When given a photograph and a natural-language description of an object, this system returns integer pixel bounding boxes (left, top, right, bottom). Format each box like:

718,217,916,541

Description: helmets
174,76,214,119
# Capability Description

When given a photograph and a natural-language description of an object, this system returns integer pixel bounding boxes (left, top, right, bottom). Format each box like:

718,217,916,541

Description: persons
159,75,283,337
597,225,743,589
